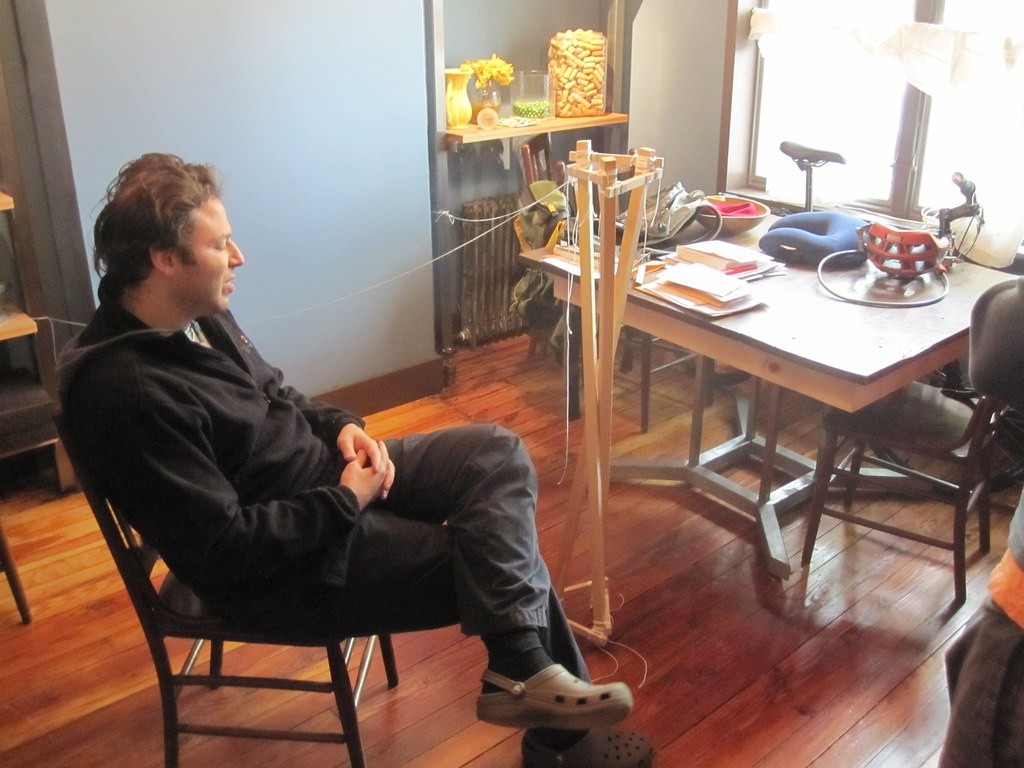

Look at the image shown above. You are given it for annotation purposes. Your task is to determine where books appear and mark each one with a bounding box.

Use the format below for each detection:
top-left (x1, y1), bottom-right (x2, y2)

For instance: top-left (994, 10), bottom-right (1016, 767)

top-left (542, 239), bottom-right (789, 316)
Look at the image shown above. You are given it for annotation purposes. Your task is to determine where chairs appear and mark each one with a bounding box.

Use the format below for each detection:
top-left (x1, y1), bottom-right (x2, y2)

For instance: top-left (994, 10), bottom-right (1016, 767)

top-left (53, 410), bottom-right (402, 768)
top-left (802, 278), bottom-right (1024, 601)
top-left (521, 132), bottom-right (715, 433)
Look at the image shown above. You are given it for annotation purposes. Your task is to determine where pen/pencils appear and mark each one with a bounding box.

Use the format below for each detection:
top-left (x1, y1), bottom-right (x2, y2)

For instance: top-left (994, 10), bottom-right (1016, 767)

top-left (722, 264), bottom-right (763, 274)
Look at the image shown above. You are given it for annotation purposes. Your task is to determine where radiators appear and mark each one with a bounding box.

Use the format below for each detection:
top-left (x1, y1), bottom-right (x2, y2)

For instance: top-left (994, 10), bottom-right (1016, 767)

top-left (456, 193), bottom-right (532, 349)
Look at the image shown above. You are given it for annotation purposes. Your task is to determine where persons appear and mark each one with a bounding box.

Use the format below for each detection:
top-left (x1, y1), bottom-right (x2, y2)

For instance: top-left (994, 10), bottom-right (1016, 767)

top-left (58, 153), bottom-right (654, 767)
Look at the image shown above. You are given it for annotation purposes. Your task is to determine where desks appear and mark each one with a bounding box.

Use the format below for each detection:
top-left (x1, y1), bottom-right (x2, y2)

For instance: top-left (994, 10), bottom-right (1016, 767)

top-left (518, 215), bottom-right (1023, 583)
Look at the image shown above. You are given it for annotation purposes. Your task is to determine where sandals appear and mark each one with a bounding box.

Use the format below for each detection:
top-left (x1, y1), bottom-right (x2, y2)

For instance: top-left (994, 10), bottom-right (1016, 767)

top-left (476, 663), bottom-right (633, 729)
top-left (522, 726), bottom-right (654, 768)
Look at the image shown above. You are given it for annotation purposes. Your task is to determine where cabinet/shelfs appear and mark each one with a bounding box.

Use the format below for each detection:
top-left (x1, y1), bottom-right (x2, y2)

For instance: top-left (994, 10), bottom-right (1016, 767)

top-left (0, 72), bottom-right (80, 492)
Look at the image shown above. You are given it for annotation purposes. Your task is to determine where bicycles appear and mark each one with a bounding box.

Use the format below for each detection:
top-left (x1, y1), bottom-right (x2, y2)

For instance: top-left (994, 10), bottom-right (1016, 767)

top-left (670, 140), bottom-right (1024, 489)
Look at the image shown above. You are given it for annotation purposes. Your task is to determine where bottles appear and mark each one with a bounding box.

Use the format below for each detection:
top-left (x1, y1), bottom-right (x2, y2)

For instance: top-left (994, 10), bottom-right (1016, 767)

top-left (445, 69), bottom-right (472, 128)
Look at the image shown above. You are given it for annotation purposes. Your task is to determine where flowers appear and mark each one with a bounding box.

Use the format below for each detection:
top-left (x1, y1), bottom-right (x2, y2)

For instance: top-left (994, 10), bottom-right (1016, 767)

top-left (460, 53), bottom-right (515, 90)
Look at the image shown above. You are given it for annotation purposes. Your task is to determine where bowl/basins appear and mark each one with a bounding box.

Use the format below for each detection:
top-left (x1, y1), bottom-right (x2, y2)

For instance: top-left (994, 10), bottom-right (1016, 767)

top-left (696, 195), bottom-right (771, 236)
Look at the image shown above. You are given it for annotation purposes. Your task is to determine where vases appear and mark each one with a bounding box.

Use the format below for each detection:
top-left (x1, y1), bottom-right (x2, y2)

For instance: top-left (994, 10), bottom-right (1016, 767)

top-left (445, 69), bottom-right (474, 130)
top-left (469, 77), bottom-right (503, 125)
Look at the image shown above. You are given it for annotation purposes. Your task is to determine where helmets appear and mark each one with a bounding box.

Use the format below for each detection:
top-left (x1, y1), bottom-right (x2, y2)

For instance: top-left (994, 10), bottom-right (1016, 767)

top-left (856, 221), bottom-right (951, 283)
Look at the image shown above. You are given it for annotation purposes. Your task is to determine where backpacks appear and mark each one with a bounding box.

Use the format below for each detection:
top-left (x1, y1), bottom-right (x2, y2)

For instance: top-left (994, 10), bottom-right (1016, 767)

top-left (510, 178), bottom-right (573, 327)
top-left (563, 182), bottom-right (722, 256)
